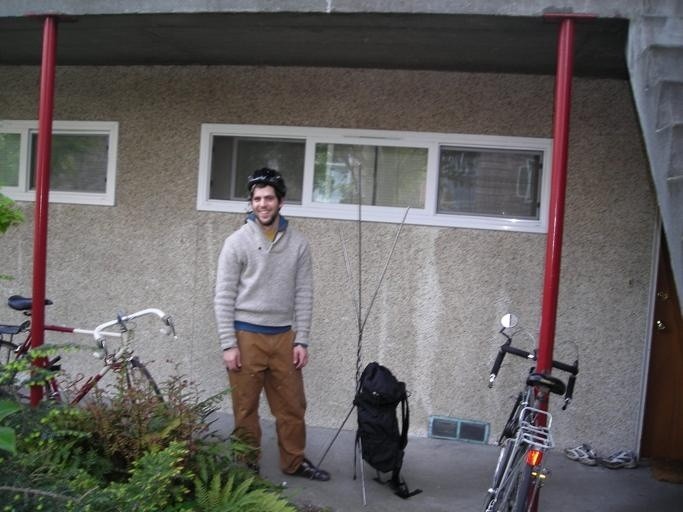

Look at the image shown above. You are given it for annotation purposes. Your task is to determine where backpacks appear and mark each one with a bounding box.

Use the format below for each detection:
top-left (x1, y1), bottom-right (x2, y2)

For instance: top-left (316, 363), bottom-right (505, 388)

top-left (353, 361), bottom-right (423, 498)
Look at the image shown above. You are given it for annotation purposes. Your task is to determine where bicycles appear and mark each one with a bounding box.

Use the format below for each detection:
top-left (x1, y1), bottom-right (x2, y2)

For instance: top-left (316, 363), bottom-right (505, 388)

top-left (0, 293), bottom-right (178, 419)
top-left (479, 314), bottom-right (581, 512)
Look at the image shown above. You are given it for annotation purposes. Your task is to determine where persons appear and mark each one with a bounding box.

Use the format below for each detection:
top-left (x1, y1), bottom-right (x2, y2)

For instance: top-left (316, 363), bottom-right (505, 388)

top-left (212, 166), bottom-right (331, 487)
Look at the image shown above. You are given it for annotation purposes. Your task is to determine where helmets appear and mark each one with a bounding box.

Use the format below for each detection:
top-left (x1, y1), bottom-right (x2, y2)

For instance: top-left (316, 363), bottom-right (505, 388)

top-left (246, 167), bottom-right (287, 198)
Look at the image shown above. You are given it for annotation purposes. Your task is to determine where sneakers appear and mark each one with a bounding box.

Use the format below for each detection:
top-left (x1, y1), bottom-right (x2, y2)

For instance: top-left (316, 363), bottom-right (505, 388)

top-left (564, 443), bottom-right (598, 467)
top-left (600, 448), bottom-right (638, 469)
top-left (289, 459), bottom-right (331, 482)
